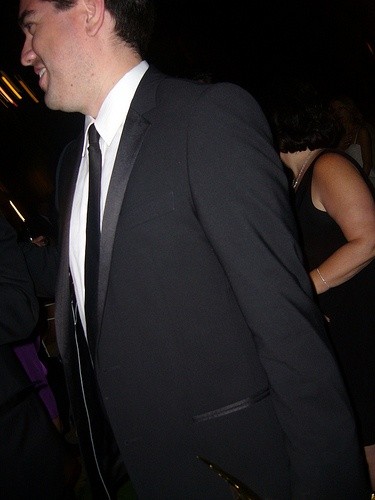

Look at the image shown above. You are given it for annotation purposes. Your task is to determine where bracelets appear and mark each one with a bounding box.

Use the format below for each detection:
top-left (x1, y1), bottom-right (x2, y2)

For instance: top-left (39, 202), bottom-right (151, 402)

top-left (317, 267), bottom-right (331, 289)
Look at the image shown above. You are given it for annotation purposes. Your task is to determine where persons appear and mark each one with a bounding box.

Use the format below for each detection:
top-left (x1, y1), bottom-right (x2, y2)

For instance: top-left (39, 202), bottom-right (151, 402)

top-left (0, 212), bottom-right (67, 500)
top-left (330, 90), bottom-right (375, 202)
top-left (23, 214), bottom-right (48, 247)
top-left (269, 79), bottom-right (375, 492)
top-left (21, 0), bottom-right (363, 500)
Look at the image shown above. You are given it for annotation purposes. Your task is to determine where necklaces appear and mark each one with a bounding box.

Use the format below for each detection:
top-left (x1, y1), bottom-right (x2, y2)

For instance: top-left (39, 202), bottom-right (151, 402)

top-left (290, 148), bottom-right (317, 189)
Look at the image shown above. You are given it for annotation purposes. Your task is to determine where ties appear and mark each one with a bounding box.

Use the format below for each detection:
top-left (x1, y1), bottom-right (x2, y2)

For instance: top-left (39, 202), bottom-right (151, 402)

top-left (84, 124), bottom-right (103, 361)
